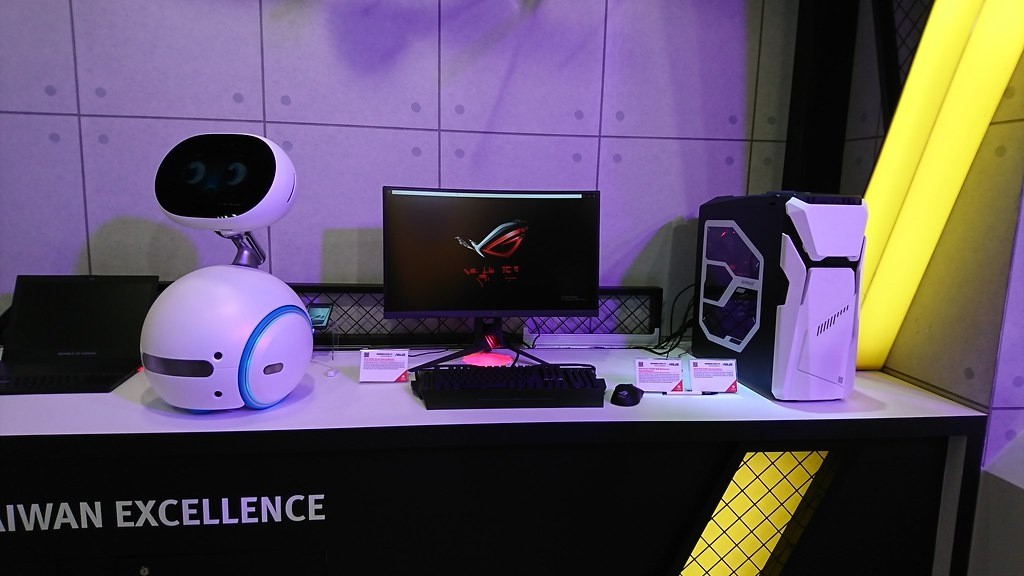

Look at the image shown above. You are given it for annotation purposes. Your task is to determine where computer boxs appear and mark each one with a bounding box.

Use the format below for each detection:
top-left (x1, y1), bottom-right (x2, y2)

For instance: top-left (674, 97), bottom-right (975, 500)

top-left (691, 191), bottom-right (868, 403)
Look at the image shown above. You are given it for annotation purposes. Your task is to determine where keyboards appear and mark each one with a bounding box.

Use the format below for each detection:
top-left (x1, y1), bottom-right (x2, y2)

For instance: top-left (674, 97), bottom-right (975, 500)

top-left (414, 368), bottom-right (604, 410)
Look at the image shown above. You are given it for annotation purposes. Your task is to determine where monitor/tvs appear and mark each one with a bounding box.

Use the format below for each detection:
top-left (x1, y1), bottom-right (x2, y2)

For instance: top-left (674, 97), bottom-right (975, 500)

top-left (382, 186), bottom-right (600, 371)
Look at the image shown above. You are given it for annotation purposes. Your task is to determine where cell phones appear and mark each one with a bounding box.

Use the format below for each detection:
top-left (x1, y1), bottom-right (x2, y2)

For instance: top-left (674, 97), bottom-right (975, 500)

top-left (308, 303), bottom-right (333, 330)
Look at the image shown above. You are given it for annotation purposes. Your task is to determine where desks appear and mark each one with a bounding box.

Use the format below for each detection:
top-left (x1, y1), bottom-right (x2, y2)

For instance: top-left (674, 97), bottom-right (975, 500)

top-left (0, 343), bottom-right (990, 576)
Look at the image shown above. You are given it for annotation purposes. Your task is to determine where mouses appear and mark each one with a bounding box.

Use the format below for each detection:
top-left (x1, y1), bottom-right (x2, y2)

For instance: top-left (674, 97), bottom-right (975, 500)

top-left (610, 384), bottom-right (645, 406)
